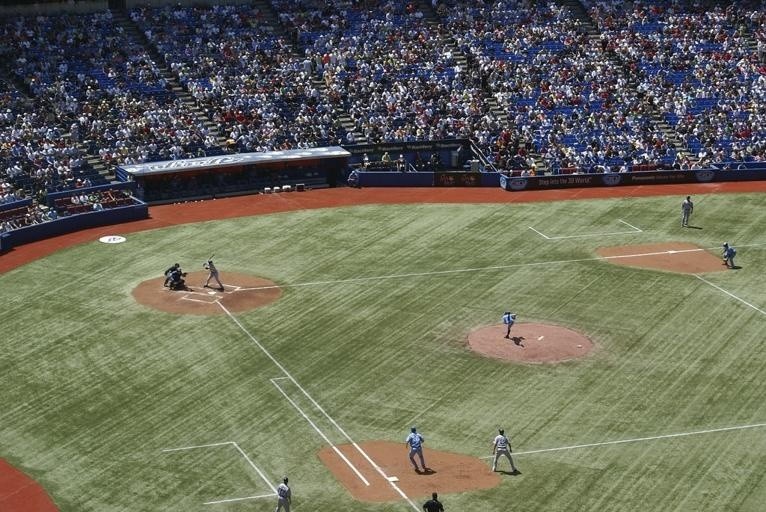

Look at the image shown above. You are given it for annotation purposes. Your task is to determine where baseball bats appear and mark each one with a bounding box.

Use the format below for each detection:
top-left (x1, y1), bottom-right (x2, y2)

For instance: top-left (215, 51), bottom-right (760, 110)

top-left (206, 254), bottom-right (215, 263)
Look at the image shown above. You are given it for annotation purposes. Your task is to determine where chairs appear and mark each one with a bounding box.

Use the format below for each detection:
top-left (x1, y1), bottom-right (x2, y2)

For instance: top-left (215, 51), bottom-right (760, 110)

top-left (1, 0), bottom-right (766, 235)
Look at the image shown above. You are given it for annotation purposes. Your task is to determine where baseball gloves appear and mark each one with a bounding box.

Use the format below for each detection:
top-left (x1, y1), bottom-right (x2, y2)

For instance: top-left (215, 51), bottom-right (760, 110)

top-left (182, 273), bottom-right (187, 277)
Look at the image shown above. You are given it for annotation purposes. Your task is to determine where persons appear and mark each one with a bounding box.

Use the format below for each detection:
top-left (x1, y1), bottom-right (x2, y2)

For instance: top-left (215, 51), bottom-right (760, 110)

top-left (164, 263), bottom-right (188, 286)
top-left (169, 267), bottom-right (187, 291)
top-left (276, 476), bottom-right (293, 512)
top-left (502, 311), bottom-right (518, 338)
top-left (422, 492), bottom-right (445, 512)
top-left (201, 260), bottom-right (224, 291)
top-left (721, 241), bottom-right (738, 270)
top-left (405, 426), bottom-right (430, 476)
top-left (492, 428), bottom-right (520, 474)
